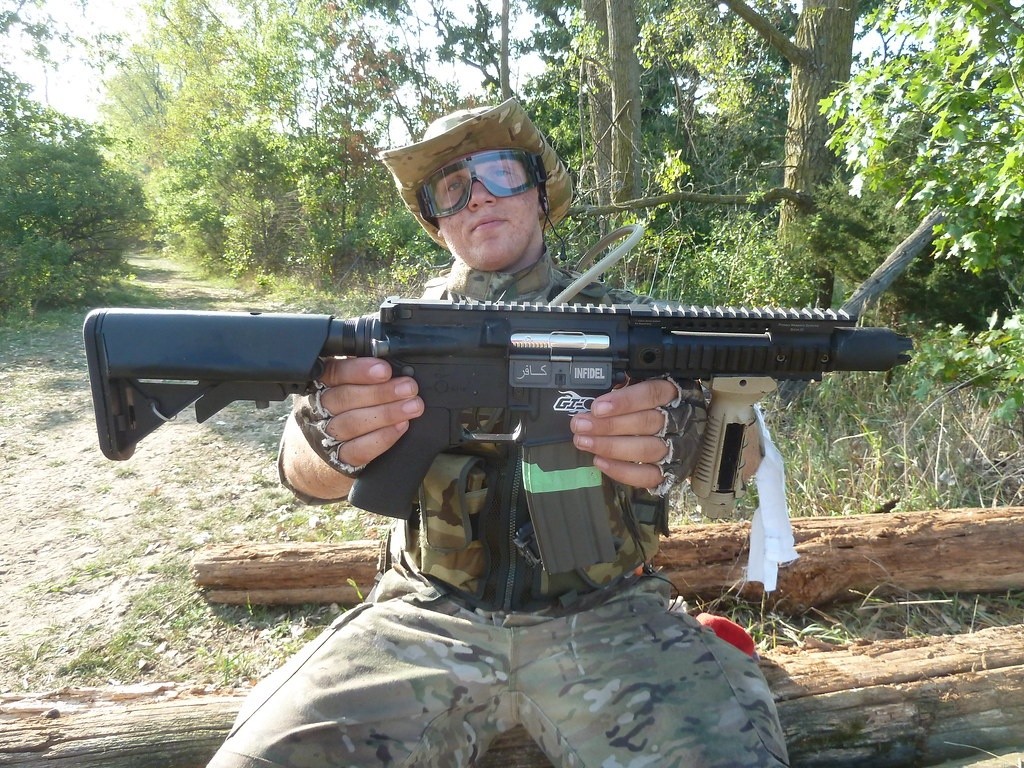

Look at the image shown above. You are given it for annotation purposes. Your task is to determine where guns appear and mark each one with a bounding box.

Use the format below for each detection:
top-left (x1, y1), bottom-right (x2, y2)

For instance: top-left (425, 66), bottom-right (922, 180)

top-left (83, 296), bottom-right (915, 577)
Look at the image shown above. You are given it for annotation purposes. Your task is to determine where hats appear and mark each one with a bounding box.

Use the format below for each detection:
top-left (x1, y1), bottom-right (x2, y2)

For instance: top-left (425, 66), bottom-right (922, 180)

top-left (377, 95), bottom-right (575, 254)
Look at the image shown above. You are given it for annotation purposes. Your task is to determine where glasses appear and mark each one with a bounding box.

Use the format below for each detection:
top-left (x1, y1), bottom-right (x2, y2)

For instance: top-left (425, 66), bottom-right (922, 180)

top-left (413, 145), bottom-right (549, 229)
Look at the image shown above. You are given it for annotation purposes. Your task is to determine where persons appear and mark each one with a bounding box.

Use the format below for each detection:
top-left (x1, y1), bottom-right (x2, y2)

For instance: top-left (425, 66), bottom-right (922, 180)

top-left (202, 97), bottom-right (790, 768)
